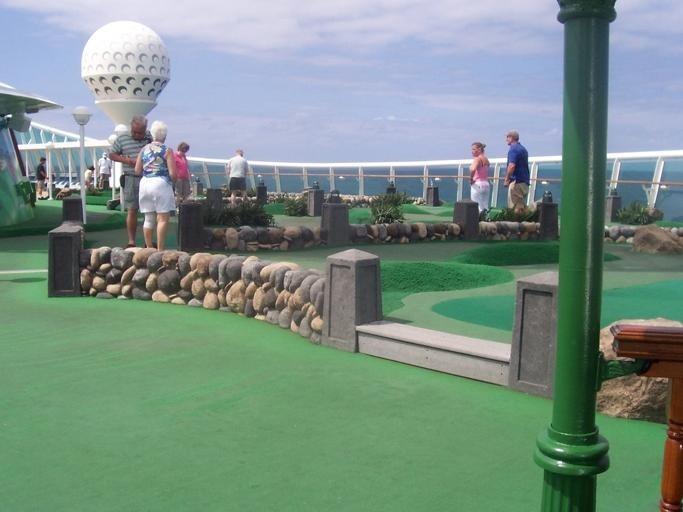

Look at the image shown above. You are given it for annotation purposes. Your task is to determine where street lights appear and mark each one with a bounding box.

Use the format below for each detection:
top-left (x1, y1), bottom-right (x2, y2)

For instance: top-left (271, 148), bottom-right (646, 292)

top-left (107, 136), bottom-right (116, 200)
top-left (46, 140), bottom-right (55, 201)
top-left (72, 107), bottom-right (92, 226)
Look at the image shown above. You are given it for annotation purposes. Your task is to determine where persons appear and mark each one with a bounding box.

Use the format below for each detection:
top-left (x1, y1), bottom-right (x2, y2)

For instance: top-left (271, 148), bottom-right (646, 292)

top-left (37, 157), bottom-right (47, 200)
top-left (470, 142), bottom-right (490, 221)
top-left (84, 151), bottom-right (112, 192)
top-left (226, 150), bottom-right (249, 203)
top-left (108, 115), bottom-right (192, 252)
top-left (504, 129), bottom-right (529, 214)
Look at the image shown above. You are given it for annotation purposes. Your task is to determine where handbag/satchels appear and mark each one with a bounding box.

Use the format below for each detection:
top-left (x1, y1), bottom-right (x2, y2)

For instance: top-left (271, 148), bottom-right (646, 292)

top-left (120, 174), bottom-right (126, 189)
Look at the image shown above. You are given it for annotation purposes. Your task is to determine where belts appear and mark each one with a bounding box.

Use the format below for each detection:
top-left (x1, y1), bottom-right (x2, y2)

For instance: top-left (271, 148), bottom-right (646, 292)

top-left (123, 173), bottom-right (141, 178)
